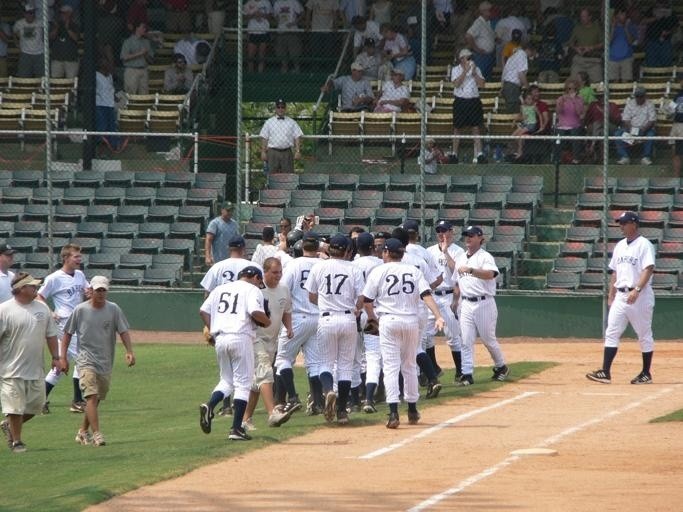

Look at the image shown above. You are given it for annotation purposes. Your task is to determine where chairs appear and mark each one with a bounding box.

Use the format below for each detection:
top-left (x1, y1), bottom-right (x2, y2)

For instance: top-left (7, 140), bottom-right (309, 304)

top-left (85, 269), bottom-right (112, 283)
top-left (9, 237), bottom-right (38, 253)
top-left (383, 191), bottom-right (414, 209)
top-left (247, 206), bottom-right (285, 223)
top-left (490, 113), bottom-right (525, 144)
top-left (668, 211), bottom-right (683, 225)
top-left (654, 258), bottom-right (679, 273)
top-left (544, 273), bottom-right (580, 290)
top-left (23, 108), bottom-right (59, 151)
top-left (60, 253), bottom-right (90, 266)
top-left (552, 257), bottom-right (587, 273)
top-left (507, 192), bottom-right (539, 235)
top-left (575, 193), bottom-right (611, 209)
top-left (31, 187), bottom-right (64, 204)
top-left (535, 80), bottom-right (565, 96)
top-left (112, 269), bottom-right (143, 285)
top-left (90, 253), bottom-right (120, 268)
top-left (407, 208), bottom-right (438, 226)
top-left (475, 225), bottom-right (493, 243)
top-left (564, 227), bottom-right (600, 244)
top-left (618, 177), bottom-right (648, 192)
top-left (648, 177), bottom-right (679, 192)
top-left (394, 111), bottom-right (427, 154)
top-left (165, 171), bottom-right (195, 187)
top-left (589, 258), bottom-right (612, 271)
top-left (469, 209), bottom-right (500, 226)
top-left (1, 109), bottom-right (26, 151)
top-left (187, 188), bottom-right (218, 218)
top-left (44, 76), bottom-right (78, 120)
top-left (10, 76), bottom-right (44, 94)
top-left (31, 92), bottom-right (69, 130)
top-left (264, 173), bottom-right (299, 189)
top-left (241, 222), bottom-right (277, 242)
top-left (107, 223), bottom-right (139, 237)
top-left (641, 65), bottom-right (676, 82)
top-left (257, 189), bottom-right (291, 206)
top-left (43, 222), bottom-right (76, 237)
top-left (658, 243), bottom-right (679, 256)
top-left (482, 176), bottom-right (512, 192)
top-left (244, 238), bottom-right (262, 255)
top-left (352, 190), bottom-right (384, 210)
top-left (0, 204), bottom-right (24, 222)
top-left (12, 222), bottom-right (44, 238)
top-left (134, 171), bottom-right (164, 185)
top-left (0, 170), bottom-right (13, 187)
top-left (391, 175), bottom-right (420, 193)
top-left (611, 194), bottom-right (642, 210)
top-left (328, 111), bottom-right (362, 154)
top-left (23, 253), bottom-right (59, 271)
top-left (22, 204), bottom-right (56, 222)
top-left (582, 177), bottom-right (617, 194)
top-left (291, 190), bottom-right (321, 208)
top-left (10, 254), bottom-right (25, 270)
top-left (408, 95), bottom-right (435, 113)
top-left (416, 192), bottom-right (444, 208)
top-left (72, 171), bottom-right (102, 187)
top-left (513, 176), bottom-right (544, 225)
top-left (132, 239), bottom-right (163, 253)
top-left (44, 171), bottom-right (73, 185)
top-left (581, 273), bottom-right (610, 287)
top-left (411, 77), bottom-right (443, 95)
top-left (642, 194), bottom-right (673, 210)
top-left (156, 95), bottom-right (190, 119)
top-left (155, 188), bottom-right (186, 204)
top-left (92, 187), bottom-right (126, 206)
top-left (493, 226), bottom-right (526, 259)
top-left (483, 112), bottom-right (491, 132)
top-left (300, 174), bottom-right (329, 190)
top-left (76, 222), bottom-right (107, 237)
top-left (662, 228), bottom-right (682, 243)
top-left (150, 110), bottom-right (180, 151)
top-left (139, 223), bottom-right (170, 239)
top-left (163, 238), bottom-right (194, 288)
top-left (362, 110), bottom-right (394, 155)
top-left (345, 208), bottom-right (375, 225)
top-left (117, 205), bottom-right (148, 221)
top-left (444, 193), bottom-right (475, 209)
top-left (426, 112), bottom-right (455, 139)
top-left (450, 175), bottom-right (481, 191)
top-left (0, 222), bottom-right (15, 238)
top-left (329, 173), bottom-right (359, 188)
top-left (607, 210), bottom-right (638, 227)
top-left (602, 80), bottom-right (636, 98)
top-left (1, 187), bottom-right (33, 204)
top-left (125, 93), bottom-right (158, 108)
top-left (178, 205), bottom-right (210, 238)
top-left (673, 194), bottom-right (683, 208)
top-left (103, 170), bottom-right (134, 186)
top-left (315, 208), bottom-right (345, 225)
top-left (118, 108), bottom-right (150, 140)
top-left (359, 175), bottom-right (390, 191)
top-left (638, 227), bottom-right (663, 243)
top-left (120, 254), bottom-right (152, 268)
top-left (486, 241), bottom-right (518, 285)
top-left (570, 210), bottom-right (603, 227)
top-left (195, 172), bottom-right (226, 204)
top-left (0, 75), bottom-right (12, 90)
top-left (376, 209), bottom-right (407, 225)
top-left (372, 225), bottom-right (398, 234)
top-left (494, 258), bottom-right (512, 287)
top-left (152, 255), bottom-right (185, 285)
top-left (601, 227), bottom-right (626, 238)
top-left (1, 92), bottom-right (36, 112)
top-left (13, 171), bottom-right (44, 188)
top-left (476, 192), bottom-right (506, 209)
top-left (37, 237), bottom-right (71, 253)
top-left (312, 225), bottom-right (338, 239)
top-left (499, 209), bottom-right (531, 252)
top-left (421, 175), bottom-right (450, 191)
top-left (144, 268), bottom-right (180, 288)
top-left (556, 243), bottom-right (593, 257)
top-left (439, 209), bottom-right (469, 225)
top-left (53, 206), bottom-right (86, 223)
top-left (61, 187), bottom-right (94, 206)
top-left (480, 95), bottom-right (498, 111)
top-left (72, 238), bottom-right (100, 253)
top-left (322, 190), bottom-right (352, 208)
top-left (171, 222), bottom-right (200, 267)
top-left (148, 206), bottom-right (179, 221)
top-left (639, 211), bottom-right (668, 229)
top-left (126, 186), bottom-right (155, 204)
top-left (340, 225), bottom-right (369, 236)
top-left (87, 206), bottom-right (116, 223)
top-left (635, 80), bottom-right (669, 99)
top-left (434, 96), bottom-right (455, 111)
top-left (594, 243), bottom-right (615, 253)
top-left (418, 63), bottom-right (449, 80)
top-left (285, 208), bottom-right (314, 224)
top-left (101, 239), bottom-right (132, 254)
top-left (651, 274), bottom-right (678, 292)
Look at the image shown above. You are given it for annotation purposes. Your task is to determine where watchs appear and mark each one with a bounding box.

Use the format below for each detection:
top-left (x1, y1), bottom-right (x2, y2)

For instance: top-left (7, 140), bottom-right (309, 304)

top-left (634, 286), bottom-right (641, 292)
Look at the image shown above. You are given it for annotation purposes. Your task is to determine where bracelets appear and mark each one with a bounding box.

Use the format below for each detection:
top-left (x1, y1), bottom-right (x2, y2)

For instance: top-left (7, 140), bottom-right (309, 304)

top-left (469, 267), bottom-right (474, 275)
top-left (52, 355), bottom-right (60, 361)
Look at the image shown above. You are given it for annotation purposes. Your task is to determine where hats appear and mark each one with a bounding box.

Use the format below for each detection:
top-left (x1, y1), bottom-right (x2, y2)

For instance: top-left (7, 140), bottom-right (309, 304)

top-left (13, 275), bottom-right (41, 288)
top-left (91, 276), bottom-right (109, 291)
top-left (238, 266), bottom-right (262, 278)
top-left (616, 212), bottom-right (639, 223)
top-left (303, 232), bottom-right (319, 242)
top-left (222, 201), bottom-right (236, 209)
top-left (403, 221), bottom-right (419, 234)
top-left (351, 62), bottom-right (365, 71)
top-left (229, 236), bottom-right (245, 247)
top-left (356, 233), bottom-right (374, 247)
top-left (0, 244), bottom-right (15, 255)
top-left (382, 238), bottom-right (402, 251)
top-left (461, 226), bottom-right (483, 236)
top-left (435, 220), bottom-right (453, 229)
top-left (23, 4), bottom-right (38, 12)
top-left (330, 235), bottom-right (348, 249)
top-left (277, 98), bottom-right (285, 105)
top-left (635, 86), bottom-right (646, 95)
top-left (365, 38), bottom-right (375, 45)
top-left (459, 49), bottom-right (472, 58)
top-left (592, 82), bottom-right (605, 95)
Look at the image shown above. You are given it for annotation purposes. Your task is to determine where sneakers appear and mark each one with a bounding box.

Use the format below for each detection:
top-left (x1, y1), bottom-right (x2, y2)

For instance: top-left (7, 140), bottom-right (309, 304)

top-left (408, 408), bottom-right (420, 425)
top-left (1, 419), bottom-right (8, 437)
top-left (386, 412), bottom-right (400, 428)
top-left (323, 391), bottom-right (336, 423)
top-left (76, 430), bottom-right (92, 444)
top-left (352, 403), bottom-right (361, 413)
top-left (200, 402), bottom-right (214, 434)
top-left (241, 418), bottom-right (257, 431)
top-left (283, 393), bottom-right (302, 415)
top-left (458, 375), bottom-right (474, 386)
top-left (642, 157), bottom-right (653, 165)
top-left (421, 365), bottom-right (444, 386)
top-left (216, 407), bottom-right (232, 417)
top-left (14, 444), bottom-right (27, 452)
top-left (426, 381), bottom-right (442, 399)
top-left (363, 399), bottom-right (375, 413)
top-left (338, 411), bottom-right (350, 423)
top-left (41, 402), bottom-right (50, 415)
top-left (229, 427), bottom-right (251, 440)
top-left (631, 372), bottom-right (652, 384)
top-left (617, 157), bottom-right (630, 165)
top-left (493, 366), bottom-right (510, 381)
top-left (587, 369), bottom-right (610, 384)
top-left (70, 399), bottom-right (85, 413)
top-left (446, 155), bottom-right (458, 164)
top-left (268, 408), bottom-right (290, 427)
top-left (477, 155), bottom-right (485, 162)
top-left (92, 432), bottom-right (105, 446)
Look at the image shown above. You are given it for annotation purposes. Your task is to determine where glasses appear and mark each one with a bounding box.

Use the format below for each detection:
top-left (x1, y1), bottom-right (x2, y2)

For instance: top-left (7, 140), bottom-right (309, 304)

top-left (281, 224), bottom-right (289, 228)
top-left (436, 228), bottom-right (447, 233)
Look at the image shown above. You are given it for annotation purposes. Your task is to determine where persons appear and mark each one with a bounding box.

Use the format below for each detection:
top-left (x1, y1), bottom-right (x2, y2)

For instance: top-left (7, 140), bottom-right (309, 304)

top-left (163, 0), bottom-right (193, 35)
top-left (201, 1), bottom-right (226, 35)
top-left (494, 6), bottom-right (529, 45)
top-left (502, 30), bottom-right (524, 64)
top-left (614, 87), bottom-right (658, 166)
top-left (609, 7), bottom-right (640, 83)
top-left (466, 1), bottom-right (503, 83)
top-left (424, 137), bottom-right (447, 165)
top-left (240, 257), bottom-right (291, 432)
top-left (584, 83), bottom-right (623, 165)
top-left (199, 266), bottom-right (271, 441)
top-left (497, 84), bottom-right (550, 166)
top-left (0, 272), bottom-right (59, 453)
top-left (274, 233), bottom-right (325, 415)
top-left (200, 234), bottom-right (265, 301)
top-left (447, 49), bottom-right (489, 164)
top-left (303, 233), bottom-right (365, 425)
top-left (119, 19), bottom-right (155, 95)
top-left (59, 278), bottom-right (135, 447)
top-left (420, 221), bottom-right (466, 386)
top-left (12, 3), bottom-right (45, 78)
top-left (567, 7), bottom-right (604, 84)
top-left (537, 27), bottom-right (566, 84)
top-left (0, 21), bottom-right (20, 78)
top-left (250, 226), bottom-right (293, 271)
top-left (241, 0), bottom-right (453, 79)
top-left (200, 236), bottom-right (264, 418)
top-left (374, 66), bottom-right (411, 113)
top-left (398, 219), bottom-right (443, 291)
top-left (349, 232), bottom-right (384, 414)
top-left (573, 72), bottom-right (598, 104)
top-left (392, 227), bottom-right (442, 399)
top-left (375, 231), bottom-right (391, 260)
top-left (586, 211), bottom-right (655, 384)
top-left (449, 226), bottom-right (511, 387)
top-left (500, 41), bottom-right (537, 114)
top-left (551, 78), bottom-right (586, 165)
top-left (0, 243), bottom-right (16, 305)
top-left (49, 5), bottom-right (80, 79)
top-left (278, 217), bottom-right (292, 249)
top-left (319, 62), bottom-right (375, 113)
top-left (36, 243), bottom-right (92, 415)
top-left (164, 52), bottom-right (193, 95)
top-left (362, 238), bottom-right (446, 428)
top-left (94, 59), bottom-right (128, 156)
top-left (259, 98), bottom-right (304, 174)
top-left (644, 5), bottom-right (678, 68)
